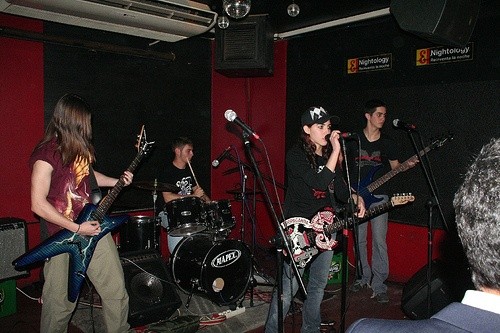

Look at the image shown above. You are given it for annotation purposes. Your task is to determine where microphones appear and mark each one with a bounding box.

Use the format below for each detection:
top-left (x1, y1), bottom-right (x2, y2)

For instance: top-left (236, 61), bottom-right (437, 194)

top-left (212, 146), bottom-right (232, 167)
top-left (393, 119), bottom-right (417, 132)
top-left (325, 133), bottom-right (352, 141)
top-left (225, 110), bottom-right (262, 141)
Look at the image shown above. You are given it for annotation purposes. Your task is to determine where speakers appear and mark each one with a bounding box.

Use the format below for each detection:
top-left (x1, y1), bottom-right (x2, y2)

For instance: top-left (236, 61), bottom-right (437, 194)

top-left (401, 258), bottom-right (470, 321)
top-left (0, 217), bottom-right (30, 281)
top-left (117, 248), bottom-right (182, 328)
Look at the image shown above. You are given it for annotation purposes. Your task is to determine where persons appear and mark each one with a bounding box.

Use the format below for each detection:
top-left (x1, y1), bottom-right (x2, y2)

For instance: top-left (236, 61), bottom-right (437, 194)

top-left (27, 91), bottom-right (134, 333)
top-left (345, 139), bottom-right (500, 333)
top-left (153, 137), bottom-right (210, 224)
top-left (264, 100), bottom-right (420, 333)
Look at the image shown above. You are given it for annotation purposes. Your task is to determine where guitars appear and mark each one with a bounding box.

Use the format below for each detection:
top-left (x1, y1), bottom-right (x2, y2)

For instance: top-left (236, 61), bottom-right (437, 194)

top-left (12, 124), bottom-right (155, 304)
top-left (279, 192), bottom-right (416, 268)
top-left (349, 131), bottom-right (454, 213)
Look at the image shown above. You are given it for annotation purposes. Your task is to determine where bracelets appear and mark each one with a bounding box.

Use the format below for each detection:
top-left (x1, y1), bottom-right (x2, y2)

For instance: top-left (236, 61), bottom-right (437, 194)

top-left (76, 224), bottom-right (80, 234)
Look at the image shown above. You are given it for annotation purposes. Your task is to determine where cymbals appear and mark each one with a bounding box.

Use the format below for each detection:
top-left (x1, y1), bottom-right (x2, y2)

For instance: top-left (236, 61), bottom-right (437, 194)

top-left (225, 188), bottom-right (262, 195)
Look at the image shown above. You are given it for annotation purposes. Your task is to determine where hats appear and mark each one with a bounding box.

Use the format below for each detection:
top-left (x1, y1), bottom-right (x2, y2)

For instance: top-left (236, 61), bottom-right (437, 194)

top-left (301, 106), bottom-right (341, 125)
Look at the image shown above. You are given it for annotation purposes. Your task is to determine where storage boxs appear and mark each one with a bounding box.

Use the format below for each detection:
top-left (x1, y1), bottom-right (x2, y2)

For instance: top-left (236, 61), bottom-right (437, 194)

top-left (0, 278), bottom-right (18, 318)
top-left (301, 252), bottom-right (347, 285)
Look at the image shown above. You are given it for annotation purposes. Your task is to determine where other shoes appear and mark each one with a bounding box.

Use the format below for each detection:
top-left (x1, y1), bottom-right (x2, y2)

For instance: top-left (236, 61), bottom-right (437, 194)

top-left (349, 281), bottom-right (367, 292)
top-left (374, 291), bottom-right (389, 303)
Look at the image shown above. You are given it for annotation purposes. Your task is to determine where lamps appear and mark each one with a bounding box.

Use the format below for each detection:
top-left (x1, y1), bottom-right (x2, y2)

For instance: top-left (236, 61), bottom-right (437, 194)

top-left (287, 0), bottom-right (300, 17)
top-left (223, 0), bottom-right (251, 19)
top-left (274, 7), bottom-right (392, 42)
top-left (218, 16), bottom-right (229, 28)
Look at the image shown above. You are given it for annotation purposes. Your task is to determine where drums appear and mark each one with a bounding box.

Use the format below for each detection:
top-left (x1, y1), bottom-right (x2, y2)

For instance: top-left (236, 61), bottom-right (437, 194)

top-left (202, 197), bottom-right (240, 235)
top-left (169, 230), bottom-right (253, 306)
top-left (164, 194), bottom-right (209, 238)
top-left (115, 216), bottom-right (163, 264)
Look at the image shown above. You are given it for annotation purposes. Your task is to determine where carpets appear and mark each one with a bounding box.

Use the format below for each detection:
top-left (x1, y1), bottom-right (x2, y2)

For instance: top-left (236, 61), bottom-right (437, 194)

top-left (70, 280), bottom-right (303, 333)
